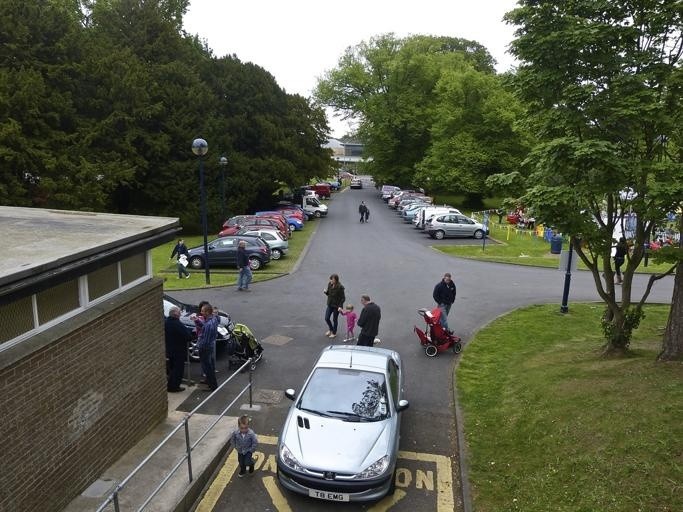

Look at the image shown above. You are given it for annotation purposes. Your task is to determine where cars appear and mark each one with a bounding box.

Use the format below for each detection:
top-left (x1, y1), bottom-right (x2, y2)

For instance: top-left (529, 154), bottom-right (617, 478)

top-left (350, 179), bottom-right (362, 189)
top-left (379, 185), bottom-right (489, 240)
top-left (275, 345), bottom-right (410, 502)
top-left (185, 182), bottom-right (340, 271)
top-left (163, 292), bottom-right (232, 361)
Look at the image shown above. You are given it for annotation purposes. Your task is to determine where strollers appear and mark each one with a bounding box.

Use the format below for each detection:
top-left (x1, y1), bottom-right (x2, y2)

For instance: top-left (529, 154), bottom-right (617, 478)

top-left (222, 323), bottom-right (262, 371)
top-left (414, 308), bottom-right (462, 357)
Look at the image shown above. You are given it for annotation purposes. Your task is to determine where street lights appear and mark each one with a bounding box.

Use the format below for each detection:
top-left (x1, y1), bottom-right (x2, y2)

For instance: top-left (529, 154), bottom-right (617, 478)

top-left (218, 157), bottom-right (228, 223)
top-left (191, 138), bottom-right (209, 283)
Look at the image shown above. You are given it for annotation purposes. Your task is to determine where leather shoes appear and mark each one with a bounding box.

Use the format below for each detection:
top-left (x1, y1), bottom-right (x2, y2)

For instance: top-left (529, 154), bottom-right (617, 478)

top-left (168, 388), bottom-right (185, 392)
top-left (200, 379), bottom-right (215, 391)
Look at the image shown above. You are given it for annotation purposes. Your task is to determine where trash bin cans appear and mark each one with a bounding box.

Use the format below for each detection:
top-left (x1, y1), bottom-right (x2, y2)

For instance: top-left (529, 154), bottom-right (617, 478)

top-left (551, 236), bottom-right (563, 254)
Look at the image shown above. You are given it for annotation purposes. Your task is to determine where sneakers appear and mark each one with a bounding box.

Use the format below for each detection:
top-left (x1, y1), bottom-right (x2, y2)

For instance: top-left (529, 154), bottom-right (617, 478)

top-left (348, 336), bottom-right (356, 341)
top-left (249, 465), bottom-right (253, 473)
top-left (236, 287), bottom-right (251, 292)
top-left (239, 467), bottom-right (247, 475)
top-left (343, 338), bottom-right (348, 342)
top-left (329, 333), bottom-right (336, 338)
top-left (186, 273), bottom-right (191, 278)
top-left (325, 330), bottom-right (332, 336)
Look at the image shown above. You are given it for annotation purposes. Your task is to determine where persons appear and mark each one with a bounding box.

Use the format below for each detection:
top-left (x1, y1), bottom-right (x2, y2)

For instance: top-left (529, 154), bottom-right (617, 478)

top-left (359, 201), bottom-right (370, 224)
top-left (236, 241), bottom-right (253, 292)
top-left (323, 274), bottom-right (345, 338)
top-left (189, 301), bottom-right (220, 392)
top-left (433, 273), bottom-right (456, 330)
top-left (613, 238), bottom-right (627, 284)
top-left (340, 305), bottom-right (359, 342)
top-left (356, 295), bottom-right (381, 347)
top-left (231, 416), bottom-right (257, 478)
top-left (169, 239), bottom-right (192, 279)
top-left (165, 307), bottom-right (195, 393)
